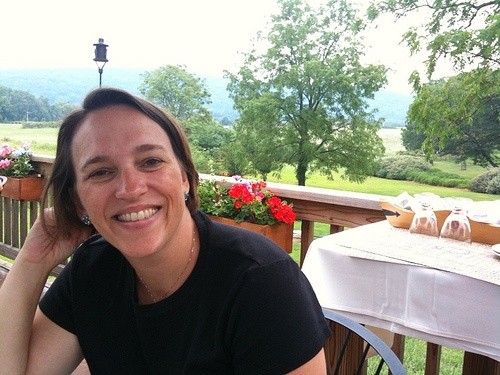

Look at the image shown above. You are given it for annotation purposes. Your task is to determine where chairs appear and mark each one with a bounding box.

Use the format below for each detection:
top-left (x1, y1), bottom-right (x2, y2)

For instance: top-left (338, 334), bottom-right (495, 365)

top-left (315, 308), bottom-right (406, 375)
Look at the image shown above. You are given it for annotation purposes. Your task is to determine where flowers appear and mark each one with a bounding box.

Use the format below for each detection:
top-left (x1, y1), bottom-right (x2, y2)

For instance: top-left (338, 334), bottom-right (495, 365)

top-left (0, 143), bottom-right (34, 177)
top-left (196, 175), bottom-right (297, 225)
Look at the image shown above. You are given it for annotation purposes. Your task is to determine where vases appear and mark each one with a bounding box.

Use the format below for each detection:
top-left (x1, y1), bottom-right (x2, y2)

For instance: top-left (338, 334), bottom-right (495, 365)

top-left (2, 175), bottom-right (45, 200)
top-left (207, 216), bottom-right (293, 256)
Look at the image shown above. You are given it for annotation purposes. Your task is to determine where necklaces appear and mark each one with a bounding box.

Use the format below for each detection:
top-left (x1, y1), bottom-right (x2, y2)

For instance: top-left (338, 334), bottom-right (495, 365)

top-left (133, 226), bottom-right (197, 304)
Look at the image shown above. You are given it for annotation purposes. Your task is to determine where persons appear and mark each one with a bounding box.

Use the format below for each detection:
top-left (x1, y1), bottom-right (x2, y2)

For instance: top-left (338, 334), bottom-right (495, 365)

top-left (0, 87), bottom-right (333, 375)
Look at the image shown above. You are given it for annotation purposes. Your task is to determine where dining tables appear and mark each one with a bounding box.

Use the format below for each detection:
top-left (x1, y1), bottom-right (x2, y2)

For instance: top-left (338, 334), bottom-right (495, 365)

top-left (301, 219), bottom-right (499, 374)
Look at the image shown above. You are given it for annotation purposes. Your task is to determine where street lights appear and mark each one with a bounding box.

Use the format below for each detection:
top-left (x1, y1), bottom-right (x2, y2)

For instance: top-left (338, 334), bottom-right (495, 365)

top-left (93, 37), bottom-right (109, 88)
